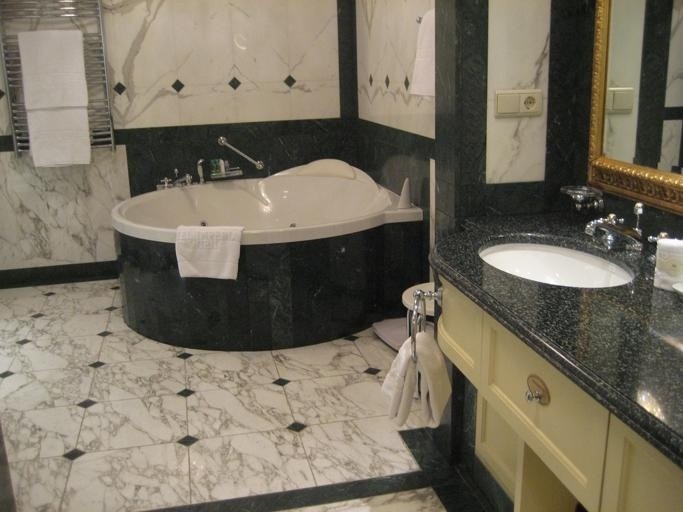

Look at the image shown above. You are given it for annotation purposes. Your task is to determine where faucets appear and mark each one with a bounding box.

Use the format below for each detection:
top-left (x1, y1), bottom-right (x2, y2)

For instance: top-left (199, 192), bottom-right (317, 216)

top-left (196, 158), bottom-right (206, 183)
top-left (583, 220), bottom-right (648, 249)
top-left (174, 173), bottom-right (194, 186)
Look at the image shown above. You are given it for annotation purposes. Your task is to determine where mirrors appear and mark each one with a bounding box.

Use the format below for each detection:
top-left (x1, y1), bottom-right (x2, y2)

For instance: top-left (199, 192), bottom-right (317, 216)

top-left (587, 1), bottom-right (681, 216)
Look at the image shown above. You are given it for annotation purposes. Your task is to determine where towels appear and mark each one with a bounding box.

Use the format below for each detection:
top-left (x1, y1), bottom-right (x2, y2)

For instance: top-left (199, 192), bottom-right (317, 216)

top-left (172, 222), bottom-right (241, 280)
top-left (382, 330), bottom-right (452, 430)
top-left (409, 7), bottom-right (434, 100)
top-left (14, 28), bottom-right (90, 168)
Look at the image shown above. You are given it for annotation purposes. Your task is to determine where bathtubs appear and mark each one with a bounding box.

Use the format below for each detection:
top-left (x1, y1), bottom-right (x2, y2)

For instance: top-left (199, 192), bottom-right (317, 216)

top-left (110, 159), bottom-right (423, 348)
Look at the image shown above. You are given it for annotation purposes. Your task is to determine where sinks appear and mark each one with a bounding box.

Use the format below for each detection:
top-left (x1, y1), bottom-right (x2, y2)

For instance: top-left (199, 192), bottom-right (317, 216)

top-left (477, 233), bottom-right (635, 290)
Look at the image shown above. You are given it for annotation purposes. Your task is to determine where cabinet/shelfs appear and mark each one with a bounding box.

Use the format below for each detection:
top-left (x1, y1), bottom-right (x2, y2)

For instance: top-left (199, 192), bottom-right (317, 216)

top-left (481, 315), bottom-right (682, 510)
